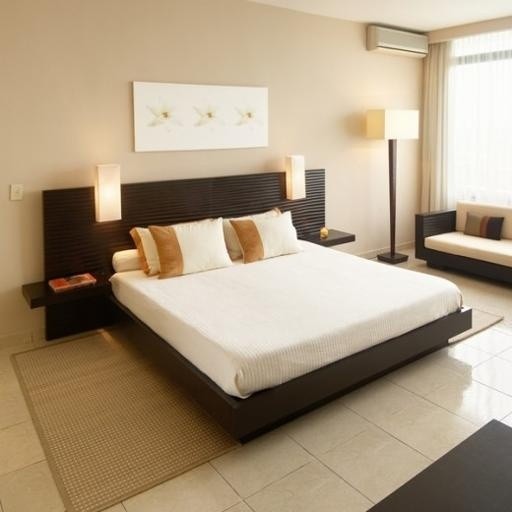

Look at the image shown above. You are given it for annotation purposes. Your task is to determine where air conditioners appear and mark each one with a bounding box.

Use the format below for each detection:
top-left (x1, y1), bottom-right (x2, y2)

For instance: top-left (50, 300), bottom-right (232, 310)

top-left (367, 25), bottom-right (428, 60)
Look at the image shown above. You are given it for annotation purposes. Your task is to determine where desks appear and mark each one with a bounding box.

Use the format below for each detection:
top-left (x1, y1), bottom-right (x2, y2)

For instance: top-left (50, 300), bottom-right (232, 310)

top-left (300, 228), bottom-right (355, 247)
top-left (21, 267), bottom-right (111, 308)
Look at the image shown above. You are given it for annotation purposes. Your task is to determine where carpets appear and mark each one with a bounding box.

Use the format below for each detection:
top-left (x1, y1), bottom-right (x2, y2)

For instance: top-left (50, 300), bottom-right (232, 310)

top-left (11, 306), bottom-right (505, 512)
top-left (365, 417), bottom-right (512, 511)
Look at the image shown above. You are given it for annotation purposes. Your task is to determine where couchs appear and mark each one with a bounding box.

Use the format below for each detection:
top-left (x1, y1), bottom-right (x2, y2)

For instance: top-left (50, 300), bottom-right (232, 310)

top-left (414, 201), bottom-right (511, 288)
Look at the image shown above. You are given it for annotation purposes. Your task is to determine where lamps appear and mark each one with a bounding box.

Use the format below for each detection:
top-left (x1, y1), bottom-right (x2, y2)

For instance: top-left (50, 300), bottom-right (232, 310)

top-left (286, 155), bottom-right (306, 202)
top-left (366, 107), bottom-right (418, 263)
top-left (94, 164), bottom-right (122, 225)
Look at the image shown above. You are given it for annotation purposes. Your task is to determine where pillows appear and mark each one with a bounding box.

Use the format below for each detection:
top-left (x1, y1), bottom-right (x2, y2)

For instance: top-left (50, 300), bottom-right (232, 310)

top-left (222, 207), bottom-right (281, 262)
top-left (127, 217), bottom-right (216, 278)
top-left (463, 211), bottom-right (504, 240)
top-left (111, 248), bottom-right (143, 272)
top-left (230, 211), bottom-right (299, 264)
top-left (148, 217), bottom-right (233, 281)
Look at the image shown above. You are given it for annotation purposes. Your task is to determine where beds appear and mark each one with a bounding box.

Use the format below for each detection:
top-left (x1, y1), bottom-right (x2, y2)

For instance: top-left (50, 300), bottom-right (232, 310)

top-left (42, 170), bottom-right (472, 444)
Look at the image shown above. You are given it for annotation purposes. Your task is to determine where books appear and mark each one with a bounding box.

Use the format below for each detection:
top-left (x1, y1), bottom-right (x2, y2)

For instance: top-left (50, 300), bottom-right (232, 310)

top-left (48, 273), bottom-right (96, 293)
